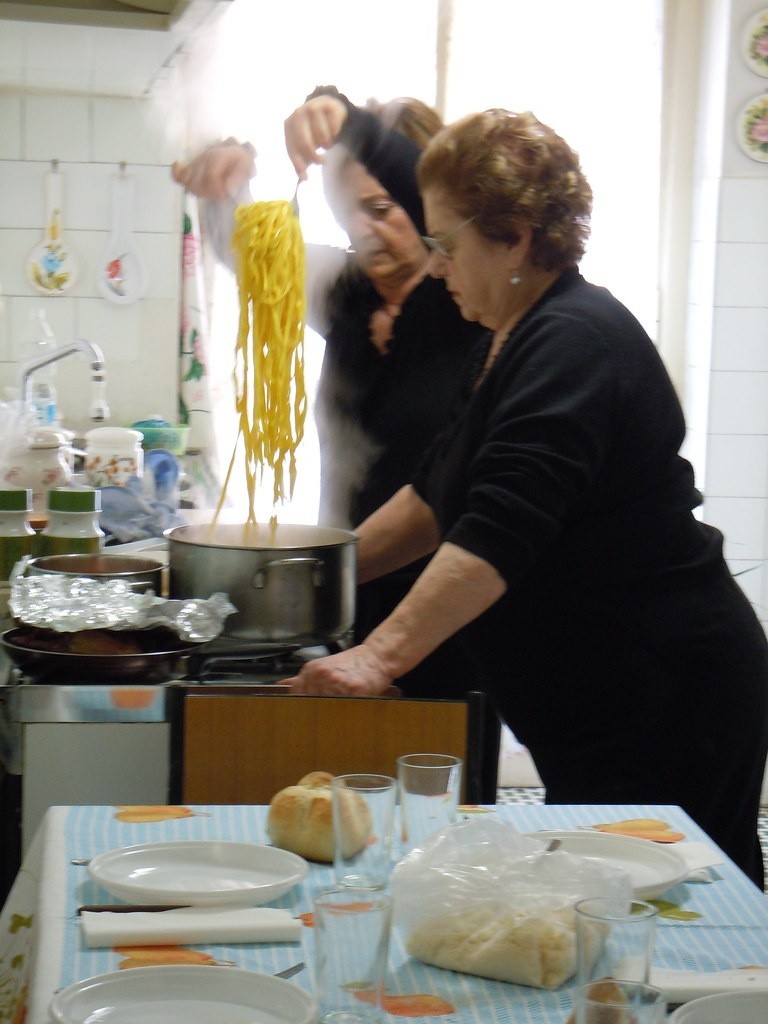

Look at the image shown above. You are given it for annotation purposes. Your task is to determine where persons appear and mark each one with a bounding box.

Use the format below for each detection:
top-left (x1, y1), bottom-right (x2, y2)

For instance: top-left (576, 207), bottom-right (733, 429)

top-left (277, 109), bottom-right (768, 892)
top-left (171, 84), bottom-right (503, 803)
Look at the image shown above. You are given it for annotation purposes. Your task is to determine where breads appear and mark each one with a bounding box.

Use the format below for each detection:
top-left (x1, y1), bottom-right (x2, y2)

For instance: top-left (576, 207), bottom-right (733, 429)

top-left (266, 770), bottom-right (372, 865)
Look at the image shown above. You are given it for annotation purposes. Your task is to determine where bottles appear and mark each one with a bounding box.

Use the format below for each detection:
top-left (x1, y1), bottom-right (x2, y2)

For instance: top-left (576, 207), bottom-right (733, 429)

top-left (0, 384), bottom-right (143, 588)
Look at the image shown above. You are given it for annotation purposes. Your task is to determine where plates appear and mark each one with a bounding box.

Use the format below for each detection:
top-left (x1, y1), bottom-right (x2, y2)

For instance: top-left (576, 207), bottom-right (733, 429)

top-left (524, 831), bottom-right (688, 901)
top-left (89, 842), bottom-right (307, 907)
top-left (48, 965), bottom-right (313, 1024)
top-left (668, 991), bottom-right (768, 1024)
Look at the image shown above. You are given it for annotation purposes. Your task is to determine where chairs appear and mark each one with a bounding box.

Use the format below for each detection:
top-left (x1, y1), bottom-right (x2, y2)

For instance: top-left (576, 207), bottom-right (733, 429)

top-left (171, 688), bottom-right (504, 805)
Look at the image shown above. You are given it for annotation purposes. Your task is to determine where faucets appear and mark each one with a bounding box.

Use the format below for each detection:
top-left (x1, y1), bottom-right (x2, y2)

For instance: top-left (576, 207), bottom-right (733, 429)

top-left (22, 341), bottom-right (110, 440)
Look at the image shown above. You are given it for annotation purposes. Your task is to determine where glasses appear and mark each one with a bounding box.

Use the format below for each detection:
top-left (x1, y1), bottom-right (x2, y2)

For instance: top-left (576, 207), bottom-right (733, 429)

top-left (419, 212), bottom-right (542, 262)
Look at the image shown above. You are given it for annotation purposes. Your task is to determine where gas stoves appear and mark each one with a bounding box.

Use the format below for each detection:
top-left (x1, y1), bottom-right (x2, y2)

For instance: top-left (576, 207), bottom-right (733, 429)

top-left (18, 601), bottom-right (364, 691)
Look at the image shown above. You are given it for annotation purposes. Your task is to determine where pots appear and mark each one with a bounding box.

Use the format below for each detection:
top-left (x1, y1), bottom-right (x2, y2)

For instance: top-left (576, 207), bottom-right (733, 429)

top-left (25, 555), bottom-right (168, 619)
top-left (0, 626), bottom-right (302, 683)
top-left (162, 523), bottom-right (360, 647)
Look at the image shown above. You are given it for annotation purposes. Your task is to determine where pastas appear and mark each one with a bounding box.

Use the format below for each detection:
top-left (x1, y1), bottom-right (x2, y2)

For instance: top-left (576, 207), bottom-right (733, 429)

top-left (198, 199), bottom-right (310, 548)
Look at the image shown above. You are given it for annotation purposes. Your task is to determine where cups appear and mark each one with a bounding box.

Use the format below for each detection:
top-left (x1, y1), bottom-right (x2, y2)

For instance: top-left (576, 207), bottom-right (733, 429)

top-left (574, 981), bottom-right (665, 1024)
top-left (331, 774), bottom-right (397, 891)
top-left (574, 898), bottom-right (660, 985)
top-left (398, 755), bottom-right (463, 856)
top-left (315, 893), bottom-right (392, 1024)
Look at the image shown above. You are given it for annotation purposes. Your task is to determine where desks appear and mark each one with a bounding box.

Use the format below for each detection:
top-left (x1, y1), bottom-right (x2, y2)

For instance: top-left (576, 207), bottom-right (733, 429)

top-left (0, 803), bottom-right (766, 1023)
